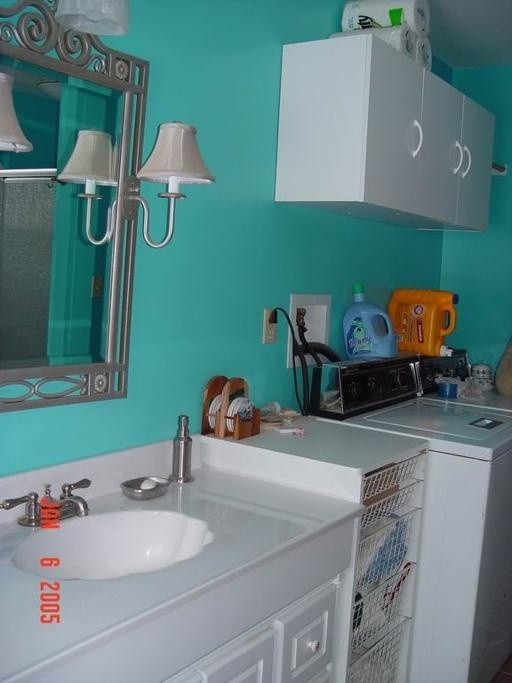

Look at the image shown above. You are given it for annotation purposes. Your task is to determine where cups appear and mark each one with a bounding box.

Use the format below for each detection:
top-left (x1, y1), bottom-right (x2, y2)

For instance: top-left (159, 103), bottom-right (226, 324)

top-left (464, 377), bottom-right (482, 396)
top-left (478, 378), bottom-right (494, 392)
top-left (471, 364), bottom-right (491, 378)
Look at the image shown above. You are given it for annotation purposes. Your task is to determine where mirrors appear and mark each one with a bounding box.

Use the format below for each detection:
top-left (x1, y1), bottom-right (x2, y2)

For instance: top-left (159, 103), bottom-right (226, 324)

top-left (0, 58), bottom-right (123, 374)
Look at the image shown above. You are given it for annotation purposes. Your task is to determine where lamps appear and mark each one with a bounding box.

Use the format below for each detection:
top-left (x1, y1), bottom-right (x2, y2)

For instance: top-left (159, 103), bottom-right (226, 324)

top-left (56, 127), bottom-right (118, 245)
top-left (124, 122), bottom-right (214, 248)
top-left (51, 0), bottom-right (130, 36)
top-left (0, 70), bottom-right (34, 153)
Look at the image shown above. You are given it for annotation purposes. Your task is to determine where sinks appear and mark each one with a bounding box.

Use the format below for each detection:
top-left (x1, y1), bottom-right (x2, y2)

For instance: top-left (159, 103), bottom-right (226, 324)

top-left (10, 511), bottom-right (213, 580)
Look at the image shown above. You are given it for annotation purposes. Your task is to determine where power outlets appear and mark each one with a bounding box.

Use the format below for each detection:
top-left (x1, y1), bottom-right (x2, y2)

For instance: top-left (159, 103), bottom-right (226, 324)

top-left (263, 309), bottom-right (276, 342)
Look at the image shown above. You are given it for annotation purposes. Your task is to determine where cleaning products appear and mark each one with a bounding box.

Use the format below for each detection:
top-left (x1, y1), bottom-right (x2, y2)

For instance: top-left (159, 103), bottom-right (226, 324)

top-left (341, 283), bottom-right (396, 358)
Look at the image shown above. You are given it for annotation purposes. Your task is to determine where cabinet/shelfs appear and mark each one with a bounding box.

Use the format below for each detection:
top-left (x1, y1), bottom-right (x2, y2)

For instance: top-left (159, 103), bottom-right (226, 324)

top-left (276, 34), bottom-right (420, 223)
top-left (421, 66), bottom-right (499, 232)
top-left (160, 572), bottom-right (355, 683)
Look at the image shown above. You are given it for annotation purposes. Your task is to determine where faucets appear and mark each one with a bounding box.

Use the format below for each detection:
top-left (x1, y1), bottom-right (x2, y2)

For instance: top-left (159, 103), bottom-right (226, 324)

top-left (1, 478), bottom-right (91, 529)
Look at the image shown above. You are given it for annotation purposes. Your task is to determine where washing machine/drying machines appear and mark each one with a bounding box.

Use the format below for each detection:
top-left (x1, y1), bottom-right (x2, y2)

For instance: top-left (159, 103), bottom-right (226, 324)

top-left (409, 351), bottom-right (511, 410)
top-left (308, 359), bottom-right (510, 683)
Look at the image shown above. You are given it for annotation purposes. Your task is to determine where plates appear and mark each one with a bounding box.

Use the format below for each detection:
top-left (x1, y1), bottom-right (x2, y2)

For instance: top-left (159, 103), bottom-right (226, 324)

top-left (207, 395), bottom-right (221, 429)
top-left (226, 396), bottom-right (255, 432)
